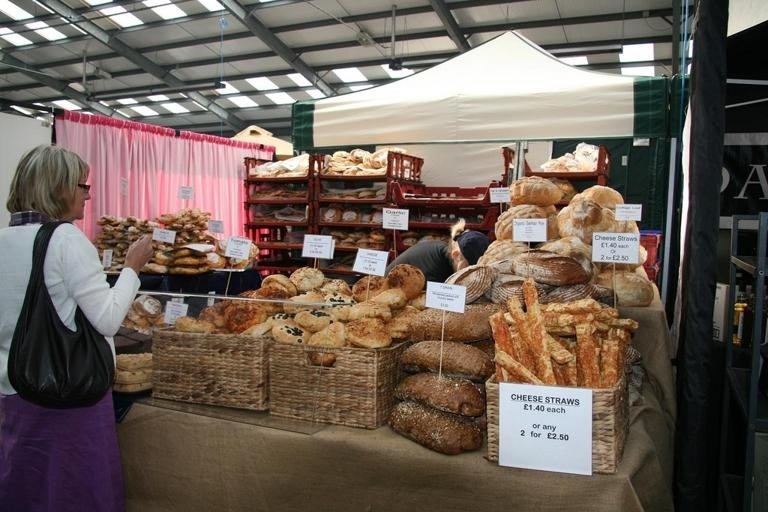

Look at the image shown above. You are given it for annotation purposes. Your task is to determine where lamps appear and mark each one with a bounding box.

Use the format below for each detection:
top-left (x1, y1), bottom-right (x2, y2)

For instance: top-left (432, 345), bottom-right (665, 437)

top-left (83, 36), bottom-right (226, 103)
top-left (389, 0), bottom-right (623, 70)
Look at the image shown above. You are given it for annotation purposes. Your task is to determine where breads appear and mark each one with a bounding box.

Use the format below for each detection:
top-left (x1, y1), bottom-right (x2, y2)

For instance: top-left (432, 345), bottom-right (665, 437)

top-left (171, 262), bottom-right (428, 366)
top-left (251, 147), bottom-right (407, 248)
top-left (110, 353), bottom-right (152, 393)
top-left (488, 277), bottom-right (640, 388)
top-left (478, 175), bottom-right (655, 307)
top-left (92, 209), bottom-right (260, 275)
top-left (119, 292), bottom-right (168, 330)
top-left (389, 264), bottom-right (495, 458)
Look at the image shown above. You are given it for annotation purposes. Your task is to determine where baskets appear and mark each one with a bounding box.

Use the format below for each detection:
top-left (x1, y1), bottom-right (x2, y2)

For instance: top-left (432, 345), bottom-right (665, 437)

top-left (267, 340), bottom-right (410, 430)
top-left (485, 368), bottom-right (630, 474)
top-left (151, 326), bottom-right (273, 410)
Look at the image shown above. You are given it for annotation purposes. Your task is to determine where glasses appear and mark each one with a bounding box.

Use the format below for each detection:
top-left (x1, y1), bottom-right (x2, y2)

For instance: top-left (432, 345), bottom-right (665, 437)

top-left (78, 184), bottom-right (90, 193)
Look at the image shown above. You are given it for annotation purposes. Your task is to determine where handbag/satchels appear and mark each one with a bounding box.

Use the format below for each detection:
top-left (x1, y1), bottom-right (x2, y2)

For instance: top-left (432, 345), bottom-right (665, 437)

top-left (8, 274), bottom-right (115, 409)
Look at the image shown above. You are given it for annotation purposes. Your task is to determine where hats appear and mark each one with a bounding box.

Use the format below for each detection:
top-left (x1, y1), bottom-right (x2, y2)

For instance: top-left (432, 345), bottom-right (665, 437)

top-left (453, 230), bottom-right (490, 265)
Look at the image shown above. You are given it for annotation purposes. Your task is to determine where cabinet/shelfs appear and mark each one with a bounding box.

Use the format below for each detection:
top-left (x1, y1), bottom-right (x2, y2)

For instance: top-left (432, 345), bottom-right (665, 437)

top-left (717, 211), bottom-right (768, 512)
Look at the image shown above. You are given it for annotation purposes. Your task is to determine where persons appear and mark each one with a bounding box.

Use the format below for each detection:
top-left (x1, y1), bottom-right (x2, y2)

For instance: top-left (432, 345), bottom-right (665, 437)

top-left (1, 146), bottom-right (159, 512)
top-left (376, 230), bottom-right (493, 289)
